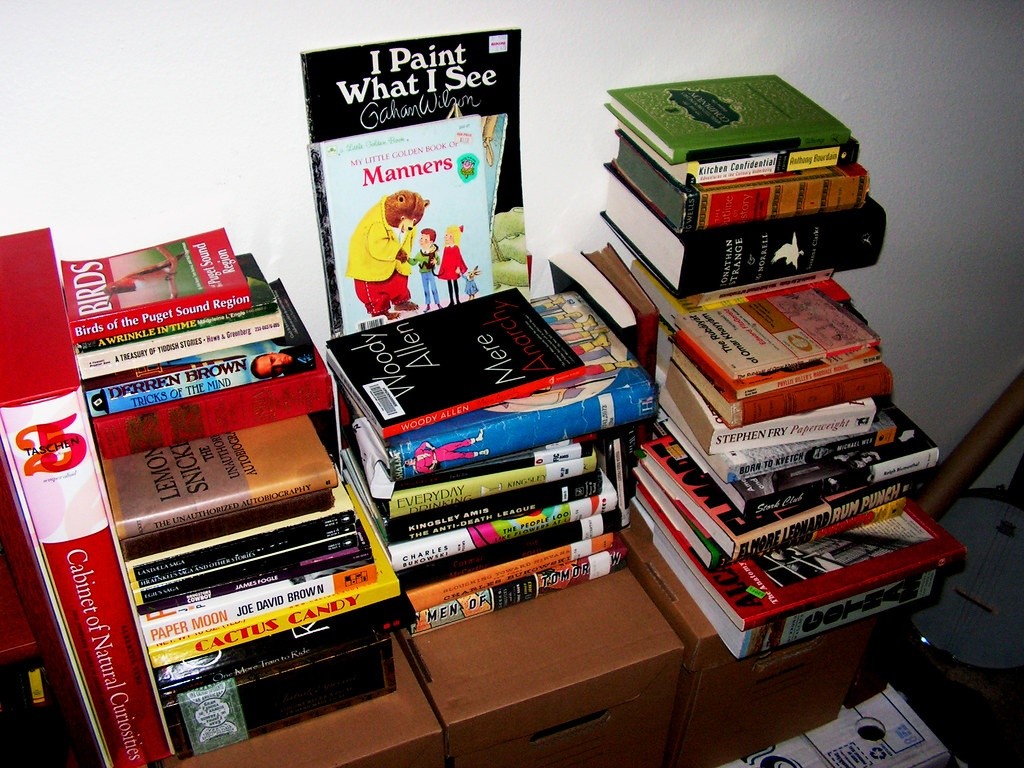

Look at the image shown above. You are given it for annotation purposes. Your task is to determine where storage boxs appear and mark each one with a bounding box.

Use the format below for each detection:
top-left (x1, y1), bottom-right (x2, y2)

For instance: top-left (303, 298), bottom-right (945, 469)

top-left (157, 497), bottom-right (949, 768)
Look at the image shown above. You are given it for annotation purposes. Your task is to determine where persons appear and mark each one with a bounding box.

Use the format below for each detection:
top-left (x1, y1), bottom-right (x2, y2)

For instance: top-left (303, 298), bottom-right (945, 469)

top-left (803, 446), bottom-right (834, 465)
top-left (849, 452), bottom-right (881, 469)
top-left (250, 344), bottom-right (317, 379)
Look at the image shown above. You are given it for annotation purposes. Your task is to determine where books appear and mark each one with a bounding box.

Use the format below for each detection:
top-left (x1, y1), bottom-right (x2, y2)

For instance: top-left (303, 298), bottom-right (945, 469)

top-left (300, 27), bottom-right (531, 340)
top-left (0, 77), bottom-right (967, 768)
